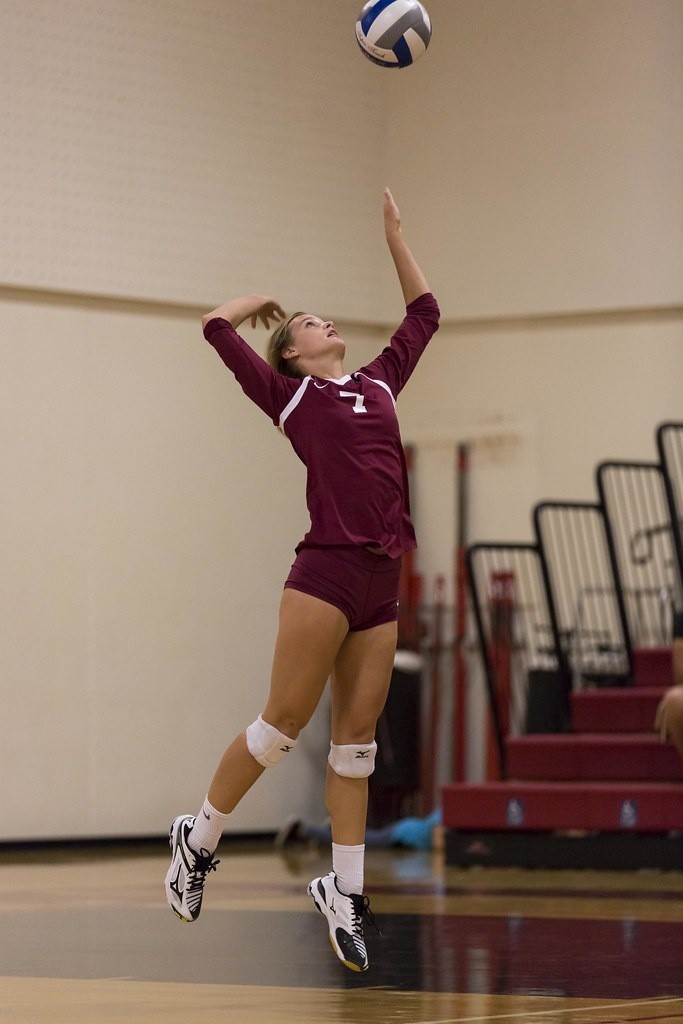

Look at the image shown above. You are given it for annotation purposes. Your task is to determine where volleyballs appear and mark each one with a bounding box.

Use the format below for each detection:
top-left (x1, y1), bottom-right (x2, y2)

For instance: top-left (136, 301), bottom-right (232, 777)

top-left (347, 0), bottom-right (436, 64)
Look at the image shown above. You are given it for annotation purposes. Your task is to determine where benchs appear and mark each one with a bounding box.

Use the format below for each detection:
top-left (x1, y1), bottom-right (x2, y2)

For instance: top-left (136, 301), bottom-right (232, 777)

top-left (441, 784), bottom-right (683, 870)
top-left (507, 734), bottom-right (683, 782)
top-left (635, 648), bottom-right (676, 685)
top-left (572, 686), bottom-right (670, 734)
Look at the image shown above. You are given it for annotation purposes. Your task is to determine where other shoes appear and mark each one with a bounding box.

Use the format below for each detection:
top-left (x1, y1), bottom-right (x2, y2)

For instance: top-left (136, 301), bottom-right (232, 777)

top-left (279, 819), bottom-right (302, 850)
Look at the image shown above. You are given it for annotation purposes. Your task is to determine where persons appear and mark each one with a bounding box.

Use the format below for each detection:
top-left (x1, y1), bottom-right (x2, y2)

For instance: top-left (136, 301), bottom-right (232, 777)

top-left (167, 189), bottom-right (440, 975)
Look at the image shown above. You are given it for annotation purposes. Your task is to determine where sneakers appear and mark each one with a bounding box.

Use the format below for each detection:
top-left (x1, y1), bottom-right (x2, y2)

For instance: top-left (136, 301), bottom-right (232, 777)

top-left (307, 871), bottom-right (373, 972)
top-left (165, 814), bottom-right (220, 922)
